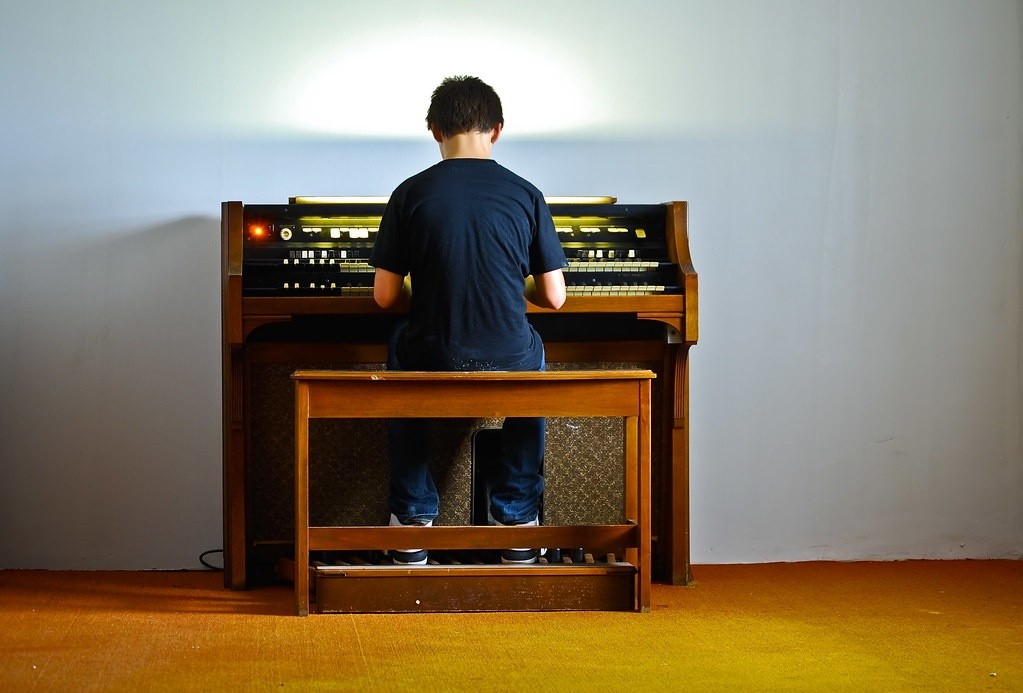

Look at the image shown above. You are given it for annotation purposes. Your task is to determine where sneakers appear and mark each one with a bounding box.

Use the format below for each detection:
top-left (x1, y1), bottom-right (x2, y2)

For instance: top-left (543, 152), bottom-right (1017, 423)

top-left (381, 511), bottom-right (434, 565)
top-left (493, 515), bottom-right (548, 564)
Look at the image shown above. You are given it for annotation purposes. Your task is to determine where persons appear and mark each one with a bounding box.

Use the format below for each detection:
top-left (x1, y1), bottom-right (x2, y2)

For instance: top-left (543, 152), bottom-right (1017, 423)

top-left (367, 75), bottom-right (569, 564)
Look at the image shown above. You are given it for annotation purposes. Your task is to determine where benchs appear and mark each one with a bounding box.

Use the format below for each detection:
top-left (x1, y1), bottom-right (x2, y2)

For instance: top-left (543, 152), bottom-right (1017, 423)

top-left (292, 368), bottom-right (658, 617)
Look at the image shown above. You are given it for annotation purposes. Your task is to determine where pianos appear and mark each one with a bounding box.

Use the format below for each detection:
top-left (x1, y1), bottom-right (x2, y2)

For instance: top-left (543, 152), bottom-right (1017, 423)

top-left (219, 193), bottom-right (700, 594)
